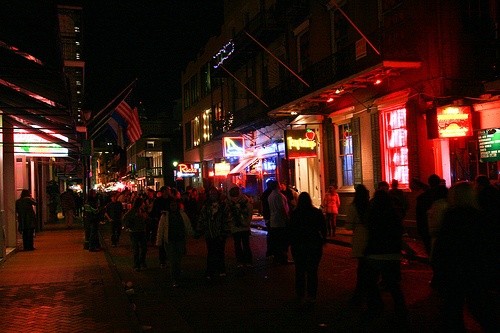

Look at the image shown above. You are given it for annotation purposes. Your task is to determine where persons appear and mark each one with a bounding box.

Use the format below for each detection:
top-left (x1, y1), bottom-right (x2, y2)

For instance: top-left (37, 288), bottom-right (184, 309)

top-left (285, 192), bottom-right (327, 305)
top-left (429, 181), bottom-right (500, 333)
top-left (322, 179), bottom-right (410, 293)
top-left (15, 176), bottom-right (300, 300)
top-left (356, 190), bottom-right (413, 330)
top-left (413, 175), bottom-right (500, 309)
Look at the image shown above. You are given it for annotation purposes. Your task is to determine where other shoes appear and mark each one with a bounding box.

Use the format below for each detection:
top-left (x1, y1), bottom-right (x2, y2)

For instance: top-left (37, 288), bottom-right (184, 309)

top-left (136, 267), bottom-right (140, 271)
top-left (297, 294), bottom-right (303, 299)
top-left (160, 264), bottom-right (163, 269)
top-left (307, 296), bottom-right (316, 302)
top-left (112, 244), bottom-right (115, 247)
top-left (143, 262), bottom-right (147, 268)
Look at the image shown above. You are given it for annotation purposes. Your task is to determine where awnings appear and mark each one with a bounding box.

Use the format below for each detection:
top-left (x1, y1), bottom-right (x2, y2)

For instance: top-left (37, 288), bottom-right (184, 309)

top-left (266, 60), bottom-right (422, 121)
top-left (215, 114), bottom-right (280, 139)
